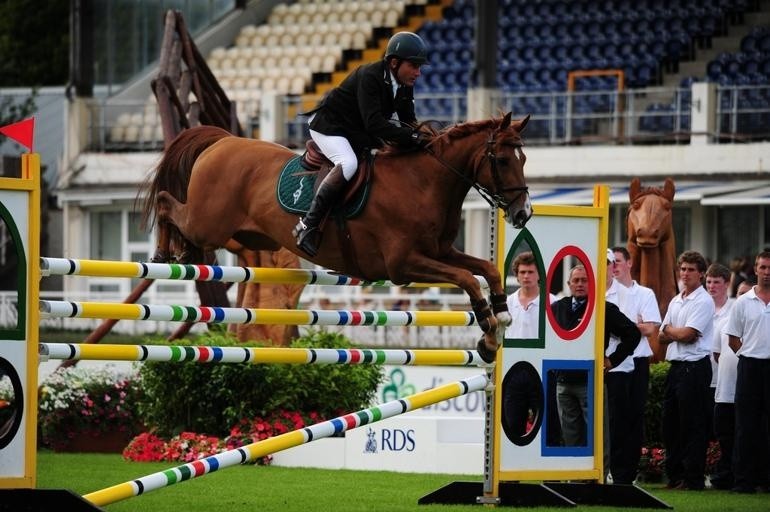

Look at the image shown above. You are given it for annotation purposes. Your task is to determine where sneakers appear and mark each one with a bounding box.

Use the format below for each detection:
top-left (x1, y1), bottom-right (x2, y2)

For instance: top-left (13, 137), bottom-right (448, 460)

top-left (659, 475), bottom-right (753, 493)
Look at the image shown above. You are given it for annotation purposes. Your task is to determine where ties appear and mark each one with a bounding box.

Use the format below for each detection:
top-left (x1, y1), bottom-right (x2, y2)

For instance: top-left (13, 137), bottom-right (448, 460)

top-left (573, 301), bottom-right (582, 311)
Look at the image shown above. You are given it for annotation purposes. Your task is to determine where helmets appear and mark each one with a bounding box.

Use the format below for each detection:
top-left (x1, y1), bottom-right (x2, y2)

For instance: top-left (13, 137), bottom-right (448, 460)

top-left (384, 31), bottom-right (431, 65)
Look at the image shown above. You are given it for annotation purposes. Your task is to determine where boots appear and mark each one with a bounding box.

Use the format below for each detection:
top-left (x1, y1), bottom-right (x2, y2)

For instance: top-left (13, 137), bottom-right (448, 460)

top-left (291, 164), bottom-right (349, 257)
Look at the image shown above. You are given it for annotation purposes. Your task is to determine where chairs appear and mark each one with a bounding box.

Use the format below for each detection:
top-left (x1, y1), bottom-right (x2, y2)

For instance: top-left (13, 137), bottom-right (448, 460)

top-left (102, 0), bottom-right (769, 151)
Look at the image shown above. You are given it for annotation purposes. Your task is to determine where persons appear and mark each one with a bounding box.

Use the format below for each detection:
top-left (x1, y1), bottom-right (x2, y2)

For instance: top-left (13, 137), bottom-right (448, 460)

top-left (603, 247), bottom-right (639, 501)
top-left (710, 280), bottom-right (754, 490)
top-left (605, 247), bottom-right (662, 484)
top-left (726, 256), bottom-right (751, 299)
top-left (503, 252), bottom-right (540, 485)
top-left (724, 252), bottom-right (770, 494)
top-left (660, 252), bottom-right (716, 491)
top-left (704, 262), bottom-right (736, 444)
top-left (546, 264), bottom-right (642, 484)
top-left (291, 32), bottom-right (429, 256)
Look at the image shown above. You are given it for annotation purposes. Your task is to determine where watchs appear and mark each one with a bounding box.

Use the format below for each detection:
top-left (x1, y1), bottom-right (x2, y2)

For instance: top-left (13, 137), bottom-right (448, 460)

top-left (662, 324), bottom-right (667, 331)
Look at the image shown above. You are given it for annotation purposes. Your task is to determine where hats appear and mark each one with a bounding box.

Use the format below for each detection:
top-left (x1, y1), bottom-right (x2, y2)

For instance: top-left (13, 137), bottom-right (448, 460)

top-left (607, 247), bottom-right (617, 268)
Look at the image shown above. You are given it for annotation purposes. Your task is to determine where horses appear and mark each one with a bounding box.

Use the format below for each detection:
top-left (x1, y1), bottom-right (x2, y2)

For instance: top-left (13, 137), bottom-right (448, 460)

top-left (625, 174), bottom-right (680, 364)
top-left (126, 108), bottom-right (535, 365)
